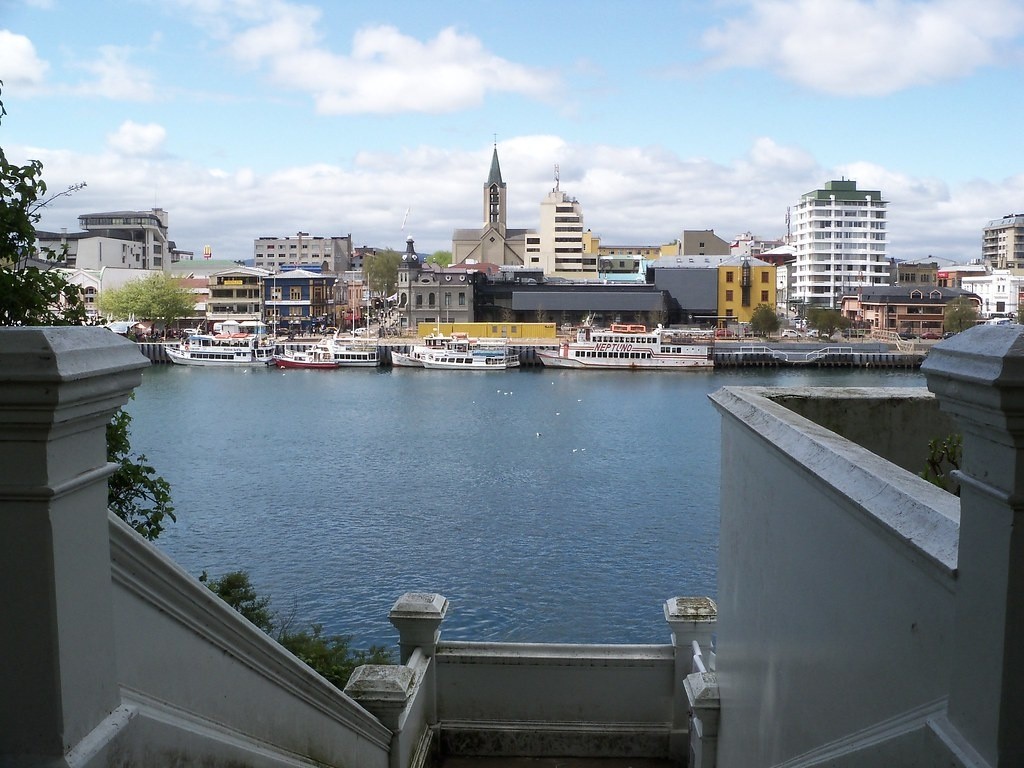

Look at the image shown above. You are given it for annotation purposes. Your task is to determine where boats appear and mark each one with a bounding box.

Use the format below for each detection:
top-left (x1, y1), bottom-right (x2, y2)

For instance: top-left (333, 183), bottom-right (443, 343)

top-left (272, 345), bottom-right (341, 370)
top-left (419, 347), bottom-right (507, 370)
top-left (165, 335), bottom-right (276, 368)
top-left (534, 323), bottom-right (715, 371)
top-left (390, 340), bottom-right (521, 368)
top-left (317, 337), bottom-right (381, 367)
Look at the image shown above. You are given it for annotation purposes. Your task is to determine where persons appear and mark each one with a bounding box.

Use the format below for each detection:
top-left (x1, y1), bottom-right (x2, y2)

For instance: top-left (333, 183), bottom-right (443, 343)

top-left (297, 325), bottom-right (320, 337)
top-left (134, 328), bottom-right (190, 346)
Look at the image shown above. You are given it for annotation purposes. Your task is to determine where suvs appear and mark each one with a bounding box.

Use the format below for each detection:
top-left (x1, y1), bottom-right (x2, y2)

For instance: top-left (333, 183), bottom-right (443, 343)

top-left (842, 327), bottom-right (866, 339)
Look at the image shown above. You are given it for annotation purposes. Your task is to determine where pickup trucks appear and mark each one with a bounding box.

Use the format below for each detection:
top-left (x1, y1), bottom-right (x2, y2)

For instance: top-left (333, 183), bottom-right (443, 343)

top-left (805, 329), bottom-right (829, 338)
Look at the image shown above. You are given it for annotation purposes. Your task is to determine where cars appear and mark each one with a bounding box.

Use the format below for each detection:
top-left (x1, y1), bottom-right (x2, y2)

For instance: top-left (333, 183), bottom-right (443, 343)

top-left (943, 332), bottom-right (955, 340)
top-left (920, 331), bottom-right (942, 340)
top-left (744, 330), bottom-right (766, 338)
top-left (350, 327), bottom-right (374, 337)
top-left (385, 327), bottom-right (398, 335)
top-left (715, 329), bottom-right (740, 338)
top-left (899, 332), bottom-right (916, 341)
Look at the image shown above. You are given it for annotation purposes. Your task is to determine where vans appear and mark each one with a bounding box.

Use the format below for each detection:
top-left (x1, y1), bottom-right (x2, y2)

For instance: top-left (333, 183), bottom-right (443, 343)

top-left (782, 329), bottom-right (802, 338)
